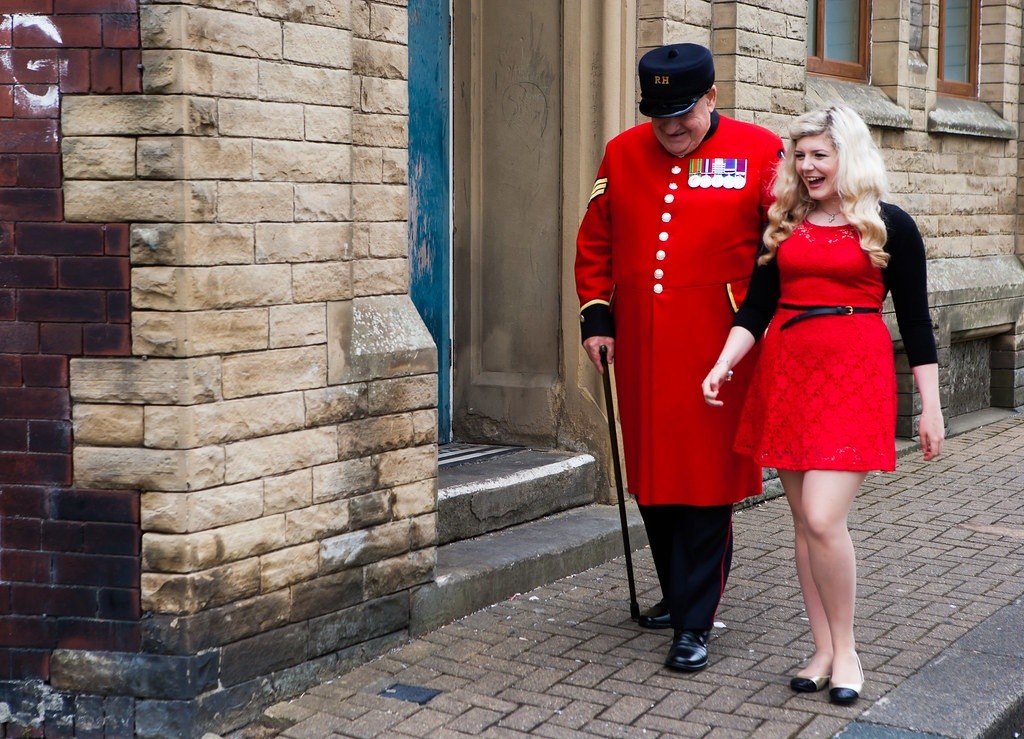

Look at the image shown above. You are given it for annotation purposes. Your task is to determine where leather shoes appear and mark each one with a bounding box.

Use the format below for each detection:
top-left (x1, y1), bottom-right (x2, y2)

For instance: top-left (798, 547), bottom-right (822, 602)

top-left (790, 669), bottom-right (830, 692)
top-left (638, 600), bottom-right (671, 629)
top-left (829, 651), bottom-right (864, 703)
top-left (665, 629), bottom-right (709, 671)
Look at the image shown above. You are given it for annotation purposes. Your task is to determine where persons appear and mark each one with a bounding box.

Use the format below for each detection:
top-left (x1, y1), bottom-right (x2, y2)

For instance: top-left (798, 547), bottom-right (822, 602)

top-left (574, 42), bottom-right (786, 673)
top-left (699, 102), bottom-right (947, 705)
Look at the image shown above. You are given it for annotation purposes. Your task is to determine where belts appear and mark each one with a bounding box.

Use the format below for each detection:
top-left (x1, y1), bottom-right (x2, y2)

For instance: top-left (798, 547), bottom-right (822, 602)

top-left (781, 303), bottom-right (879, 330)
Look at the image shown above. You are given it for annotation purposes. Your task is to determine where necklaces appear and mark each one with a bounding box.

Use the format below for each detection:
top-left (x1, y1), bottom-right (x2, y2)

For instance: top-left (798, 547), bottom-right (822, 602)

top-left (821, 206), bottom-right (842, 223)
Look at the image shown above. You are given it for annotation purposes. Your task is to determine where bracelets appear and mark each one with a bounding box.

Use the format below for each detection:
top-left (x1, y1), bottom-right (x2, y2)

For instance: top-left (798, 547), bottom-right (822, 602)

top-left (717, 358), bottom-right (733, 381)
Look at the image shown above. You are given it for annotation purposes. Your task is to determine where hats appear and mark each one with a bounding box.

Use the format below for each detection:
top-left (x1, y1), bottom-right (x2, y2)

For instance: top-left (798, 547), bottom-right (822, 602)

top-left (639, 42), bottom-right (714, 118)
